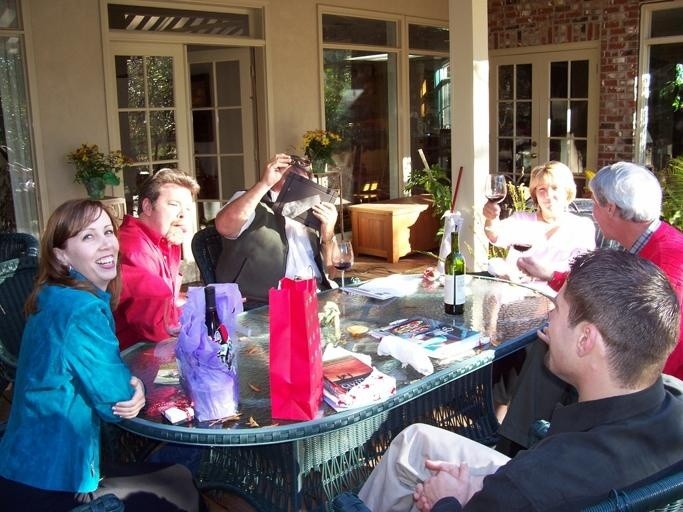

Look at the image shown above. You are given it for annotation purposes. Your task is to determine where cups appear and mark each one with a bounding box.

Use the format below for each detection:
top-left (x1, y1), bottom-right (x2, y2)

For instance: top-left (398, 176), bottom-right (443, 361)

top-left (164, 298), bottom-right (191, 339)
top-left (331, 239), bottom-right (355, 298)
top-left (511, 210), bottom-right (538, 283)
top-left (485, 172), bottom-right (507, 231)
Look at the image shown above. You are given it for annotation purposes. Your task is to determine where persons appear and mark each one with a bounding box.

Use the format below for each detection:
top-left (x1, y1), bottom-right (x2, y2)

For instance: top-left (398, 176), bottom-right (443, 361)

top-left (483, 162), bottom-right (595, 298)
top-left (216, 153), bottom-right (338, 309)
top-left (1, 199), bottom-right (199, 512)
top-left (589, 160), bottom-right (682, 378)
top-left (357, 251), bottom-right (682, 510)
top-left (103, 167), bottom-right (198, 351)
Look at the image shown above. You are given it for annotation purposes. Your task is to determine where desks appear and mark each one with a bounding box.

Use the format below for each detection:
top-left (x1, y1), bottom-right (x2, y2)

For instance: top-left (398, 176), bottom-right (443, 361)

top-left (350, 193), bottom-right (442, 264)
top-left (108, 271), bottom-right (557, 512)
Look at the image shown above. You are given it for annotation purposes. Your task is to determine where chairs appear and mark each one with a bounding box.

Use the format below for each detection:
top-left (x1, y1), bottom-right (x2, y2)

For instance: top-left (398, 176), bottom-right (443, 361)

top-left (568, 197), bottom-right (613, 252)
top-left (580, 371), bottom-right (682, 512)
top-left (0, 257), bottom-right (43, 384)
top-left (192, 227), bottom-right (222, 312)
top-left (2, 233), bottom-right (40, 260)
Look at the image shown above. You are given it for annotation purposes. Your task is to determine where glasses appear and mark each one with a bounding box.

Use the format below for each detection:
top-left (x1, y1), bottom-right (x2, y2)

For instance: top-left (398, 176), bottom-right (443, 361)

top-left (289, 156), bottom-right (311, 168)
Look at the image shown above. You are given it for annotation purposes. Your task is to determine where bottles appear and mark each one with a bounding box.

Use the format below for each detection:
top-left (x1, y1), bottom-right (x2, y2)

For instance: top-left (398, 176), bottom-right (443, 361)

top-left (444, 232), bottom-right (465, 315)
top-left (201, 286), bottom-right (221, 339)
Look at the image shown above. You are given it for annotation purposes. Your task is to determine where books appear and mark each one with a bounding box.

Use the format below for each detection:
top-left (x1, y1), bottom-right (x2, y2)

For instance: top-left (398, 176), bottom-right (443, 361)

top-left (339, 281), bottom-right (410, 300)
top-left (373, 315), bottom-right (488, 359)
top-left (322, 355), bottom-right (395, 401)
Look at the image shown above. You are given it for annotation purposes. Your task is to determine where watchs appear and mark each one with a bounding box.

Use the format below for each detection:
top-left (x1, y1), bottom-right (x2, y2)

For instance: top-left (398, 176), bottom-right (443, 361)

top-left (321, 236), bottom-right (337, 244)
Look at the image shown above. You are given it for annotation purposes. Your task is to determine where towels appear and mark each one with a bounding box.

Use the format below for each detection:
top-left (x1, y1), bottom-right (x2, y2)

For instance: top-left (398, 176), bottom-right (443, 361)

top-left (376, 334), bottom-right (435, 378)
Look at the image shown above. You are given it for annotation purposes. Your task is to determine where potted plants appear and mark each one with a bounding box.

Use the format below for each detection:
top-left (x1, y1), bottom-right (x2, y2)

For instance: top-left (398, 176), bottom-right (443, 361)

top-left (66, 143), bottom-right (134, 228)
top-left (302, 130), bottom-right (345, 188)
